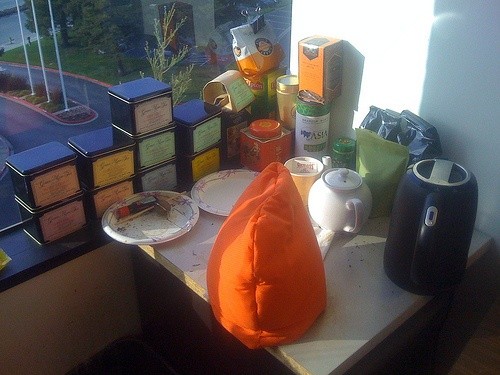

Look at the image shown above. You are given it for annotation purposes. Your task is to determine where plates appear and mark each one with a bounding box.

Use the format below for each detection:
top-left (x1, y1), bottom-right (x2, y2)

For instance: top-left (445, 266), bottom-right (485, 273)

top-left (191, 169), bottom-right (263, 217)
top-left (101, 191), bottom-right (200, 245)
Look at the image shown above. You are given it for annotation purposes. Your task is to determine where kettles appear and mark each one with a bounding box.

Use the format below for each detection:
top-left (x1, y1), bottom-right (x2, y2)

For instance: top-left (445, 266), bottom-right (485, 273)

top-left (383, 157), bottom-right (478, 296)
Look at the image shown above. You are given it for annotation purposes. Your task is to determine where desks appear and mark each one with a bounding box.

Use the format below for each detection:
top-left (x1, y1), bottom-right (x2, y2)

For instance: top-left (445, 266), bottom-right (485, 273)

top-left (138, 216), bottom-right (492, 375)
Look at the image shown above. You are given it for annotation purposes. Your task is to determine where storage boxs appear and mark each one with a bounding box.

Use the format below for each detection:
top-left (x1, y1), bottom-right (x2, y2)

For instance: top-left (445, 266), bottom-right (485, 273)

top-left (4, 36), bottom-right (344, 248)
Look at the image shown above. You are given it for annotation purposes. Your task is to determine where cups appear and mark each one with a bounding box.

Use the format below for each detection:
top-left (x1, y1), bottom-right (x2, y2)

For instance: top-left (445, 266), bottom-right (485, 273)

top-left (284, 156), bottom-right (323, 206)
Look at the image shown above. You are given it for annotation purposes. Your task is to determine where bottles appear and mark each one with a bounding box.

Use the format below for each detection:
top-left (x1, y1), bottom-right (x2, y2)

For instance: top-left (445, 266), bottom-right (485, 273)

top-left (332, 138), bottom-right (359, 170)
top-left (237, 119), bottom-right (297, 169)
top-left (295, 96), bottom-right (333, 160)
top-left (275, 75), bottom-right (302, 130)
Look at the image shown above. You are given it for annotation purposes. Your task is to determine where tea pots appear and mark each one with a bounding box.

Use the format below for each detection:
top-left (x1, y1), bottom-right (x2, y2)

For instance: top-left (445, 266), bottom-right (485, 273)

top-left (308, 156), bottom-right (373, 234)
